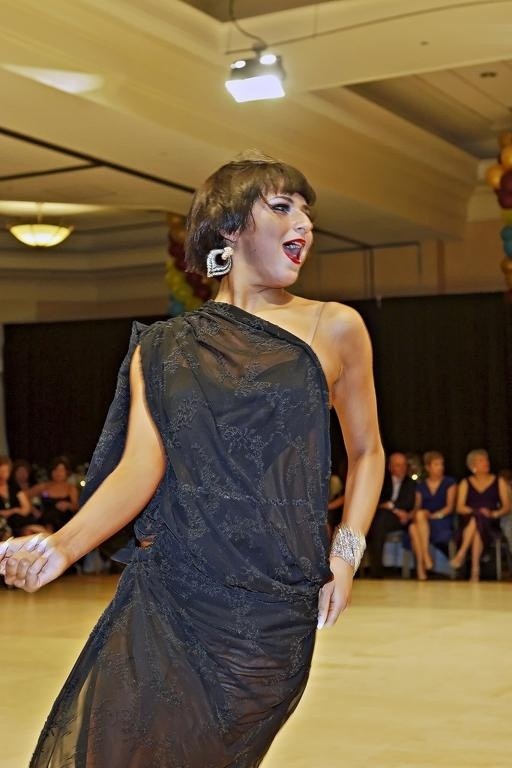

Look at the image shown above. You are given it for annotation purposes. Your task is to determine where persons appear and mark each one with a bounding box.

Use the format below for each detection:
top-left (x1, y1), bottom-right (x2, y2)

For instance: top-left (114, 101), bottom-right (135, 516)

top-left (327, 450), bottom-right (512, 583)
top-left (1, 452), bottom-right (82, 542)
top-left (0, 149), bottom-right (387, 768)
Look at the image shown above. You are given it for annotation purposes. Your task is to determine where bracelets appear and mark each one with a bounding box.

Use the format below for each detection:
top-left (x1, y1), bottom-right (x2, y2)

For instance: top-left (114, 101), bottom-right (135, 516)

top-left (326, 523), bottom-right (368, 576)
